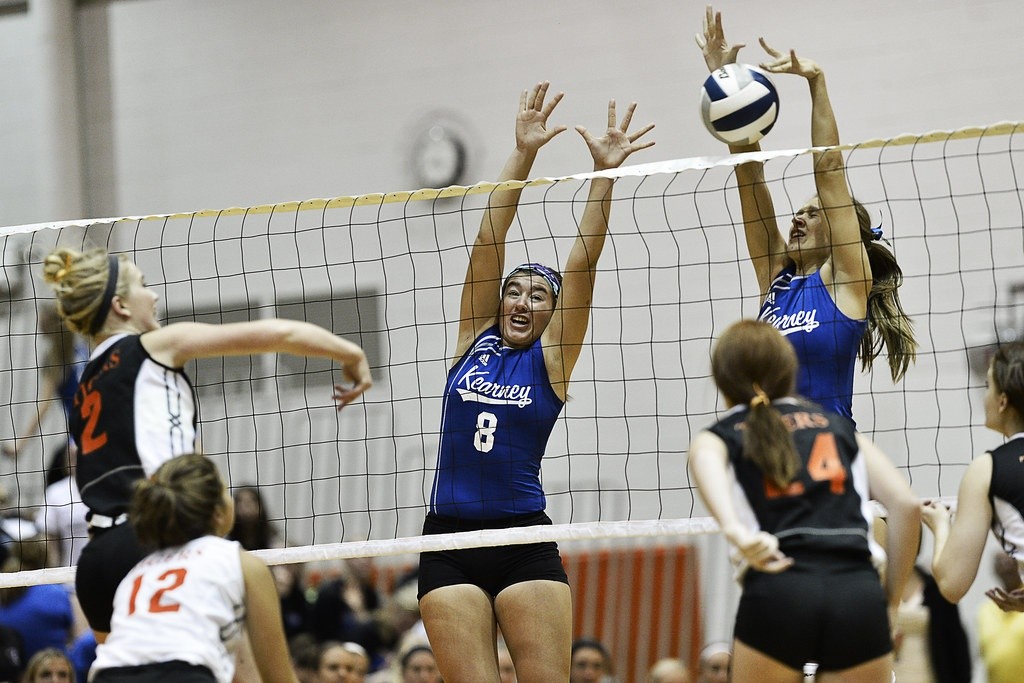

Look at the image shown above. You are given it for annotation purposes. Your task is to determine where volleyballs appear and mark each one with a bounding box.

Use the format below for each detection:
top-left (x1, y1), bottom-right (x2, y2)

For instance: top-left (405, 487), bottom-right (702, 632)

top-left (699, 59), bottom-right (783, 151)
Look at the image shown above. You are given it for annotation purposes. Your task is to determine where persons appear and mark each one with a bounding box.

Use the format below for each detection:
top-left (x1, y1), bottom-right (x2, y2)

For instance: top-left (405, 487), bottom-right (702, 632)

top-left (84, 449), bottom-right (302, 683)
top-left (872, 336), bottom-right (1024, 683)
top-left (413, 77), bottom-right (658, 683)
top-left (692, 2), bottom-right (920, 427)
top-left (491, 636), bottom-right (735, 683)
top-left (41, 242), bottom-right (374, 646)
top-left (691, 320), bottom-right (924, 683)
top-left (0, 432), bottom-right (442, 683)
